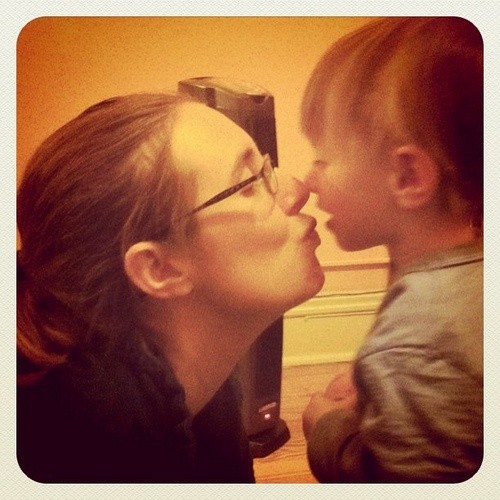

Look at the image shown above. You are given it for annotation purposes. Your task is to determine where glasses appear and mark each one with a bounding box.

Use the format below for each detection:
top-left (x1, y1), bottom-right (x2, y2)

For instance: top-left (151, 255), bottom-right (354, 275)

top-left (151, 153), bottom-right (278, 240)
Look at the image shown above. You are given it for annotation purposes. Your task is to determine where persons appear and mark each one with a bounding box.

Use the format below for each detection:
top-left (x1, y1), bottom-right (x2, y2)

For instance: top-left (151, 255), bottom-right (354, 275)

top-left (17, 93), bottom-right (325, 484)
top-left (300, 17), bottom-right (483, 483)
